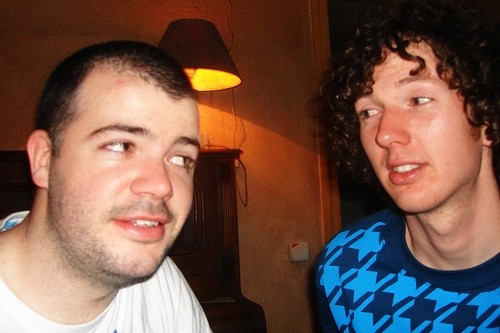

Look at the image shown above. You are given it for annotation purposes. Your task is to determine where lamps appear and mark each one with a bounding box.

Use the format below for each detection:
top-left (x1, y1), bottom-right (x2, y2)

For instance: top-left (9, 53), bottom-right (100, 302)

top-left (156, 18), bottom-right (242, 94)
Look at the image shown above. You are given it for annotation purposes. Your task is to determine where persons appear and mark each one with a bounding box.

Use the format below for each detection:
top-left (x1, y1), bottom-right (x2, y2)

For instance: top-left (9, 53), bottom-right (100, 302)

top-left (0, 41), bottom-right (213, 333)
top-left (308, 0), bottom-right (499, 333)
